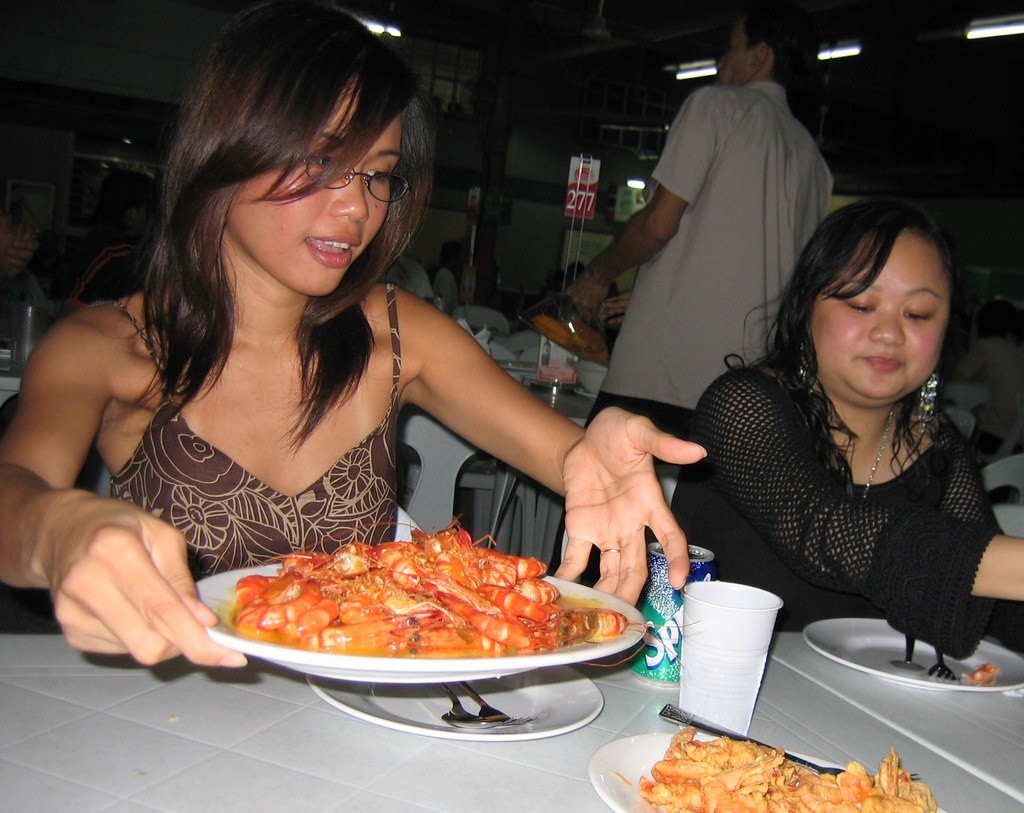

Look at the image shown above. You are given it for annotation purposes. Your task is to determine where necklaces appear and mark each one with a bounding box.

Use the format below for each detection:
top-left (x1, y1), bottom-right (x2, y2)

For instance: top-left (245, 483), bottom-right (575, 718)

top-left (856, 409), bottom-right (894, 501)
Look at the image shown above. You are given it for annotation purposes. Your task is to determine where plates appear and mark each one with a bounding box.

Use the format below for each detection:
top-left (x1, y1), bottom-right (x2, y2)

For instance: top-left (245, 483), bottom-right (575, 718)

top-left (589, 733), bottom-right (862, 813)
top-left (573, 388), bottom-right (597, 402)
top-left (0, 338), bottom-right (11, 356)
top-left (803, 617), bottom-right (1024, 692)
top-left (195, 563), bottom-right (646, 683)
top-left (299, 666), bottom-right (604, 742)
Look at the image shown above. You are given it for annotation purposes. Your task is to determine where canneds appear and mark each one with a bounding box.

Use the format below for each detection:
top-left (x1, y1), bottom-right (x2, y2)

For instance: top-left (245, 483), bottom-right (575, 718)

top-left (625, 543), bottom-right (715, 689)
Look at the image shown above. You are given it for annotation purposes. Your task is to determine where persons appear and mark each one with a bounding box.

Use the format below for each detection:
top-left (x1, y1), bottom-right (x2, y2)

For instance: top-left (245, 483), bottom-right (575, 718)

top-left (546, 0), bottom-right (833, 609)
top-left (0, 0), bottom-right (710, 667)
top-left (67, 168), bottom-right (585, 315)
top-left (670, 195), bottom-right (1024, 660)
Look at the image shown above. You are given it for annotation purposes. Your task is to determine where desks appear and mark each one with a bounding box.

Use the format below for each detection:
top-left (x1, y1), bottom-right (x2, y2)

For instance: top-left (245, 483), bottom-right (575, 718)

top-left (459, 382), bottom-right (591, 570)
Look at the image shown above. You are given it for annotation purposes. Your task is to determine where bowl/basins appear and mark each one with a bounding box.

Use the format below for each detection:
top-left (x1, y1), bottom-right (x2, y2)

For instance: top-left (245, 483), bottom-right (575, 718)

top-left (572, 364), bottom-right (608, 395)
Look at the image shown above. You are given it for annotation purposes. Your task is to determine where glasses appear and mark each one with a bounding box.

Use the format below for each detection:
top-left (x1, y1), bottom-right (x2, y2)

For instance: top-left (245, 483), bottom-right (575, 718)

top-left (297, 154), bottom-right (413, 201)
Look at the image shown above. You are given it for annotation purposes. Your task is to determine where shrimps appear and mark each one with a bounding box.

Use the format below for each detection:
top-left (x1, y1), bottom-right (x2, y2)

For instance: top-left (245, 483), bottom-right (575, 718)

top-left (231, 506), bottom-right (630, 653)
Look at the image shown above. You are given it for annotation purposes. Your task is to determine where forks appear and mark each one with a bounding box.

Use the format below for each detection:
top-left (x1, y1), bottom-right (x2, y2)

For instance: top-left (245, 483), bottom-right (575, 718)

top-left (659, 703), bottom-right (921, 782)
top-left (456, 682), bottom-right (534, 726)
top-left (928, 645), bottom-right (961, 681)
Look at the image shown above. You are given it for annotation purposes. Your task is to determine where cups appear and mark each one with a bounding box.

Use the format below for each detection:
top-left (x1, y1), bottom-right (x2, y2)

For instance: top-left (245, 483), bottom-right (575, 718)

top-left (677, 581), bottom-right (784, 736)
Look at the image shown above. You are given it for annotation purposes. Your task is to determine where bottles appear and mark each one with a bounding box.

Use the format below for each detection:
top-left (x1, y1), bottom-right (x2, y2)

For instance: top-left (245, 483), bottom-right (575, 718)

top-left (11, 304), bottom-right (42, 377)
top-left (541, 338), bottom-right (551, 365)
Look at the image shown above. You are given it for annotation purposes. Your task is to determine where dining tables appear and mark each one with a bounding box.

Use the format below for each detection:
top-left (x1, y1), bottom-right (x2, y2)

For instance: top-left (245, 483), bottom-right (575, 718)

top-left (0, 632), bottom-right (1024, 813)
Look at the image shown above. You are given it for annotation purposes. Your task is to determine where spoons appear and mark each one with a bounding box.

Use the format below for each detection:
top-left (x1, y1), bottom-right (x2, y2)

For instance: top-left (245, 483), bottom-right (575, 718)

top-left (439, 683), bottom-right (501, 730)
top-left (889, 634), bottom-right (925, 671)
